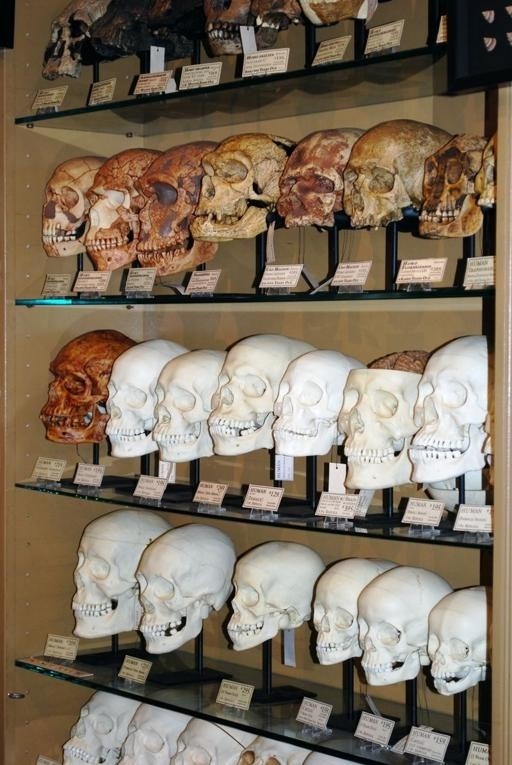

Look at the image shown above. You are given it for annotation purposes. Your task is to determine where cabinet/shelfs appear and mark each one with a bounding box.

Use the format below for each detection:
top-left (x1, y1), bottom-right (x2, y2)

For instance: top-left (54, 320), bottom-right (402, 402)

top-left (4, 22), bottom-right (511, 764)
